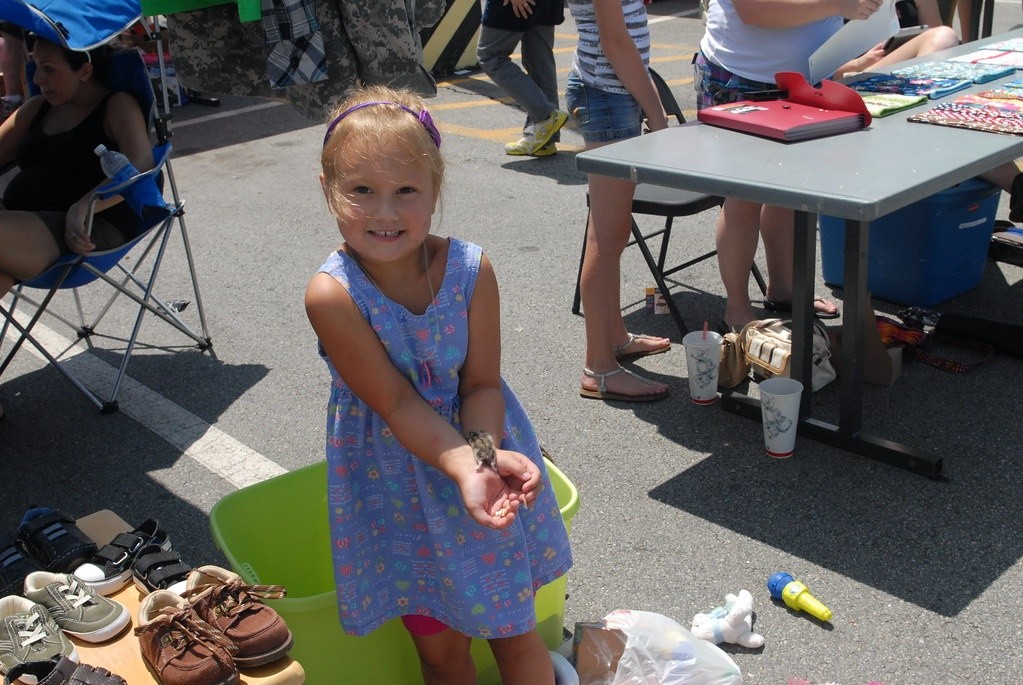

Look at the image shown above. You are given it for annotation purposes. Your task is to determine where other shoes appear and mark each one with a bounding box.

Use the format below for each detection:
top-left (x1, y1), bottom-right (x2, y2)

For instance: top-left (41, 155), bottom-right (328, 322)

top-left (1009, 172), bottom-right (1023, 222)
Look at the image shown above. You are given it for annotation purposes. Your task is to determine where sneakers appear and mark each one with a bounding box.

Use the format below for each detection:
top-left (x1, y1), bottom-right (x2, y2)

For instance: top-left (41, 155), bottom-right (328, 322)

top-left (135, 562), bottom-right (294, 685)
top-left (73, 516), bottom-right (173, 596)
top-left (1, 507), bottom-right (99, 600)
top-left (25, 571), bottom-right (131, 643)
top-left (504, 134), bottom-right (557, 156)
top-left (526, 108), bottom-right (569, 155)
top-left (0, 593), bottom-right (80, 685)
top-left (132, 543), bottom-right (193, 599)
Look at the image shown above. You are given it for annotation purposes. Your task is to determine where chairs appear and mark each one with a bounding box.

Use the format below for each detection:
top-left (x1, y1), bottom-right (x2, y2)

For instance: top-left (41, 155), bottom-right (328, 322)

top-left (571, 67), bottom-right (767, 340)
top-left (0, 0), bottom-right (208, 420)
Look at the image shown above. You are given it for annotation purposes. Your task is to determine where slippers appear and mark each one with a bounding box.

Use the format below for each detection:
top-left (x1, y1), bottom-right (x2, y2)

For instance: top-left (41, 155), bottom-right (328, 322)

top-left (763, 295), bottom-right (840, 317)
top-left (719, 320), bottom-right (733, 334)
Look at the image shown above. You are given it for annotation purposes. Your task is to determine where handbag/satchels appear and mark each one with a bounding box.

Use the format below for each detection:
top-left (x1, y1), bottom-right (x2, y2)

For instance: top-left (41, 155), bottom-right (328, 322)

top-left (719, 319), bottom-right (762, 387)
top-left (744, 318), bottom-right (837, 392)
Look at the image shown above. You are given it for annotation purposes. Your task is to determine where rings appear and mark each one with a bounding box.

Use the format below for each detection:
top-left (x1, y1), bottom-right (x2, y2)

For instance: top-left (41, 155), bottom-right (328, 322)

top-left (524, 3), bottom-right (528, 6)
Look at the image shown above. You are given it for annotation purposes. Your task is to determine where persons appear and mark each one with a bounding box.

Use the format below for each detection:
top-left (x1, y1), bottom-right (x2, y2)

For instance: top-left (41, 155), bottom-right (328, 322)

top-left (565, 2), bottom-right (672, 400)
top-left (476, 0), bottom-right (569, 157)
top-left (0, 36), bottom-right (166, 300)
top-left (305, 85), bottom-right (574, 685)
top-left (691, 0), bottom-right (1023, 340)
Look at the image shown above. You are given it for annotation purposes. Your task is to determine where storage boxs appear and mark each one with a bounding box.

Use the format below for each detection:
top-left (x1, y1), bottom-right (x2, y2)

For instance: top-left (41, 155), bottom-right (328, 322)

top-left (211, 436), bottom-right (576, 685)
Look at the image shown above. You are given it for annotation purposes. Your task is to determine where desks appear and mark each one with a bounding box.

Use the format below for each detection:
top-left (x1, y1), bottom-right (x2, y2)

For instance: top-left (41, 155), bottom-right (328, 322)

top-left (574, 29), bottom-right (1023, 480)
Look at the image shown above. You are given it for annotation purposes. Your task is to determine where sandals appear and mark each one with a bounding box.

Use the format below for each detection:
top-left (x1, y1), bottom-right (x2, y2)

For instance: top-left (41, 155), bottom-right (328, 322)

top-left (579, 361), bottom-right (669, 401)
top-left (613, 332), bottom-right (671, 357)
top-left (3, 652), bottom-right (127, 685)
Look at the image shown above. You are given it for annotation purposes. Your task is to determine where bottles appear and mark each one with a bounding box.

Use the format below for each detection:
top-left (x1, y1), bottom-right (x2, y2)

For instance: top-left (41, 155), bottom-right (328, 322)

top-left (93, 144), bottom-right (141, 184)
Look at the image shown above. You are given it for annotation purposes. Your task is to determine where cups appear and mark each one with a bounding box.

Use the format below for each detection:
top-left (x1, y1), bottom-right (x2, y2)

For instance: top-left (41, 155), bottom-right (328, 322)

top-left (757, 378), bottom-right (805, 459)
top-left (681, 330), bottom-right (726, 405)
